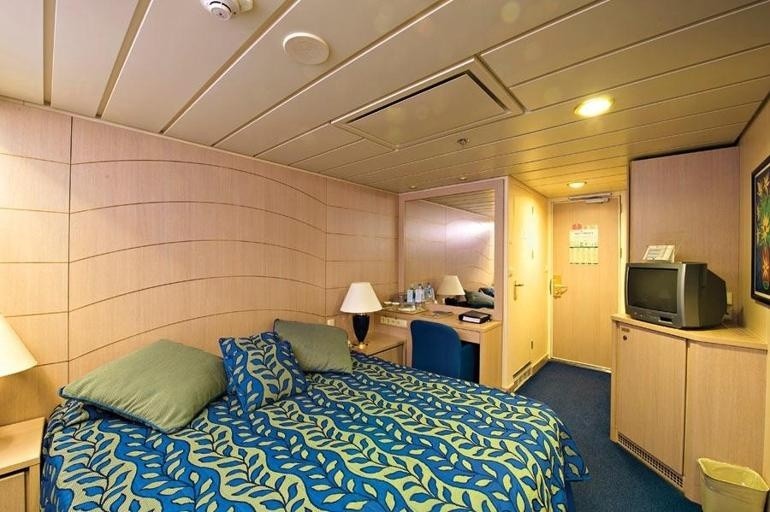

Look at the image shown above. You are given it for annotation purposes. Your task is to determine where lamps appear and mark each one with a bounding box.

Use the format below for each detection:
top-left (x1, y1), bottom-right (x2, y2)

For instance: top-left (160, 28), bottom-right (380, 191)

top-left (340, 282), bottom-right (382, 349)
top-left (437, 274), bottom-right (465, 305)
top-left (0, 313), bottom-right (37, 378)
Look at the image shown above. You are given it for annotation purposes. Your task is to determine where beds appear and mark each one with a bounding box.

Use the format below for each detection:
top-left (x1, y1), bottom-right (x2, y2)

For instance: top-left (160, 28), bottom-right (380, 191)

top-left (40, 348), bottom-right (590, 512)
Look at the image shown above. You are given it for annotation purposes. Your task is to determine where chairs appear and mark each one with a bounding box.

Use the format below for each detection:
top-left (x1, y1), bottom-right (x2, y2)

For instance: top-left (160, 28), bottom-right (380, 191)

top-left (411, 320), bottom-right (475, 381)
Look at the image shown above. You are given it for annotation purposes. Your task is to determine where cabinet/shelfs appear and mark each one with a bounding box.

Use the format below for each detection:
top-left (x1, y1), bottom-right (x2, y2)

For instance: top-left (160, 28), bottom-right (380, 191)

top-left (609, 312), bottom-right (770, 505)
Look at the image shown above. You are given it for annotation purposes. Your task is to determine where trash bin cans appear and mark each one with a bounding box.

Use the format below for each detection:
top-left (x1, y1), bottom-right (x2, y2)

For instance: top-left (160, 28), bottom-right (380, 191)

top-left (697, 457), bottom-right (770, 512)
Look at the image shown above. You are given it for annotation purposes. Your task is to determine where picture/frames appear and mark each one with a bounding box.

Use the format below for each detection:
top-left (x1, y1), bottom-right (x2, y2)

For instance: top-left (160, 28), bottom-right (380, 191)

top-left (751, 155), bottom-right (770, 305)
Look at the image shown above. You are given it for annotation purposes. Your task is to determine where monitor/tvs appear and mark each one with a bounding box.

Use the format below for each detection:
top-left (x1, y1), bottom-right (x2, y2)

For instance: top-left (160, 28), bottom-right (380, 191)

top-left (623, 261), bottom-right (728, 328)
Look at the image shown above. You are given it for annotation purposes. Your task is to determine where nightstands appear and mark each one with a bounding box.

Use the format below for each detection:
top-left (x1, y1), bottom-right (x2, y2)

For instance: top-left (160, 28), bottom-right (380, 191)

top-left (349, 331), bottom-right (407, 365)
top-left (0, 417), bottom-right (45, 512)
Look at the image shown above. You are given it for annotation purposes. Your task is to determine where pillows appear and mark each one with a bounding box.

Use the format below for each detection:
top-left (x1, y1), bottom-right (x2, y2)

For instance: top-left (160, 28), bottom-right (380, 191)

top-left (478, 288), bottom-right (494, 297)
top-left (464, 289), bottom-right (493, 307)
top-left (273, 318), bottom-right (355, 377)
top-left (219, 331), bottom-right (280, 395)
top-left (58, 340), bottom-right (227, 436)
top-left (225, 341), bottom-right (306, 413)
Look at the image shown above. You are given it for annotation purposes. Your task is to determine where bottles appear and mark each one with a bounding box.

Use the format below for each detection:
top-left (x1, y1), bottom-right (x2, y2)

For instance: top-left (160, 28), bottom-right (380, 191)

top-left (383, 282), bottom-right (435, 311)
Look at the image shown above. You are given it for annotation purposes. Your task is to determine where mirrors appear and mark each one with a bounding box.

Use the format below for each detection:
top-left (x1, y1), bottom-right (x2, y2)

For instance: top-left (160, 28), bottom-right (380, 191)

top-left (398, 176), bottom-right (503, 320)
